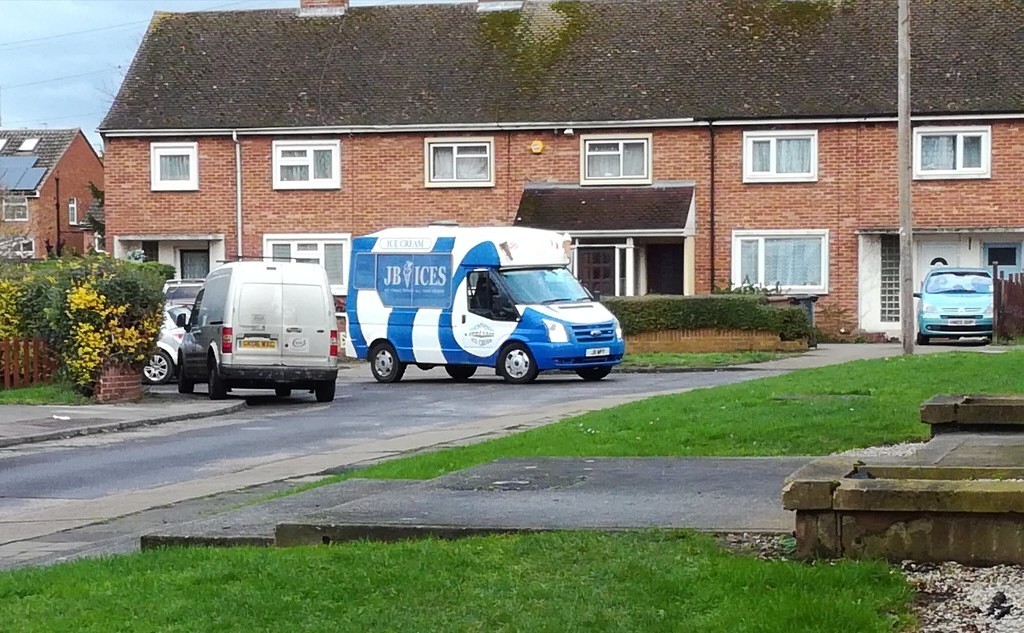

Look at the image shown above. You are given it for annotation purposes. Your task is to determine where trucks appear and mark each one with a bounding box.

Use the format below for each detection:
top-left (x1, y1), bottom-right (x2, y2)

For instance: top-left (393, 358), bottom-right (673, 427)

top-left (172, 257), bottom-right (338, 402)
top-left (344, 227), bottom-right (626, 385)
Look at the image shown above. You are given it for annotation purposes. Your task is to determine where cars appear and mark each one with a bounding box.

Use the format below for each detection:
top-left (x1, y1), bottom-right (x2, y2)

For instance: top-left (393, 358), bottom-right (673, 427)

top-left (912, 266), bottom-right (994, 345)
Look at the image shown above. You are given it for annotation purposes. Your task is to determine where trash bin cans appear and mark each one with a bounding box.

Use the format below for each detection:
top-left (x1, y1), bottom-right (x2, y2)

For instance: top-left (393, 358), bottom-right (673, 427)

top-left (788, 294), bottom-right (819, 328)
top-left (335, 312), bottom-right (346, 355)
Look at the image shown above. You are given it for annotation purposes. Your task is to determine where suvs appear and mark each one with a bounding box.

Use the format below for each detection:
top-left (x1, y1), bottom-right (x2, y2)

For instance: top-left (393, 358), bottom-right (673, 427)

top-left (141, 277), bottom-right (206, 385)
